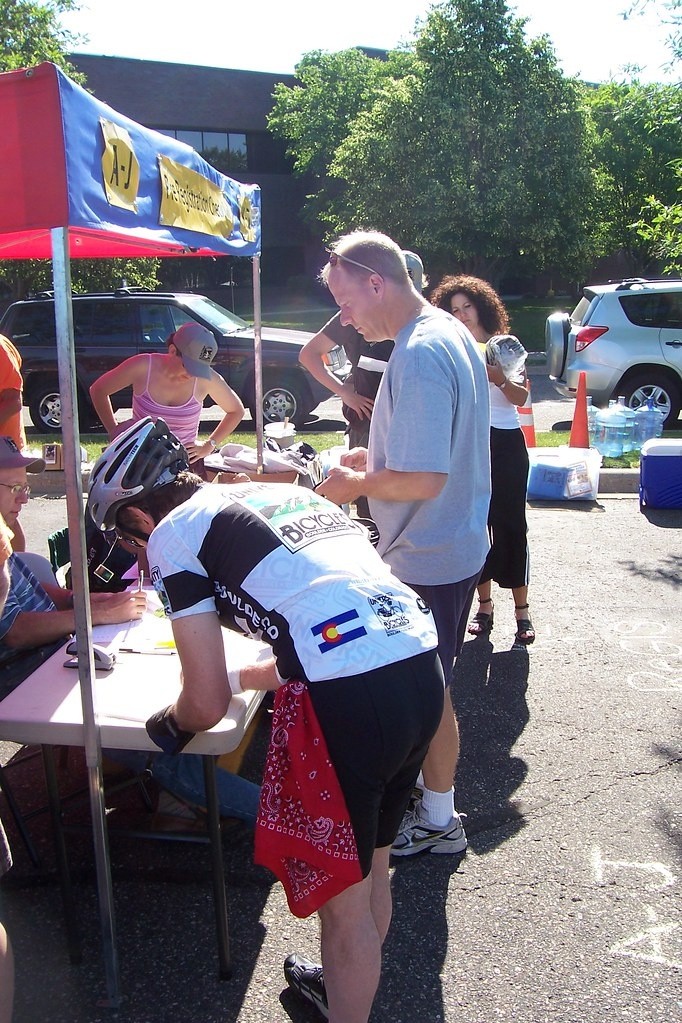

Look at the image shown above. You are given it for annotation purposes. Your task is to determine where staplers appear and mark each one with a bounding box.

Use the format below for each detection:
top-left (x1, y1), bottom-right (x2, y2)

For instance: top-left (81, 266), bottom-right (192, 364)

top-left (63, 641), bottom-right (116, 671)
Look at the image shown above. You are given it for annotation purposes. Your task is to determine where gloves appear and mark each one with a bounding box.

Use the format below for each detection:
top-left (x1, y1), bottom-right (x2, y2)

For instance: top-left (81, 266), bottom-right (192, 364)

top-left (146, 705), bottom-right (196, 757)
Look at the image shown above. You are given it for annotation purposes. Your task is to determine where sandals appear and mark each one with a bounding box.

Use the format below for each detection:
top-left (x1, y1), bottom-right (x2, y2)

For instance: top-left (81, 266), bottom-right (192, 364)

top-left (515, 603), bottom-right (534, 642)
top-left (468, 597), bottom-right (494, 633)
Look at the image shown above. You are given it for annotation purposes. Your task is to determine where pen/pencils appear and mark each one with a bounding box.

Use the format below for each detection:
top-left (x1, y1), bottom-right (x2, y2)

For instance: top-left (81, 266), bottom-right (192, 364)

top-left (138, 569), bottom-right (143, 592)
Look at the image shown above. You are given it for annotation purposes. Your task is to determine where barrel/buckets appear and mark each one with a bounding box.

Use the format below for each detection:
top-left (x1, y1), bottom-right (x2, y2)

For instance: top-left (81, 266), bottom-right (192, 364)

top-left (264, 422), bottom-right (296, 449)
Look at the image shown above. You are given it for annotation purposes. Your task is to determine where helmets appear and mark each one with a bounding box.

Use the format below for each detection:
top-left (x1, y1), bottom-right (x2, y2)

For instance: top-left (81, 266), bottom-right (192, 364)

top-left (88, 416), bottom-right (190, 530)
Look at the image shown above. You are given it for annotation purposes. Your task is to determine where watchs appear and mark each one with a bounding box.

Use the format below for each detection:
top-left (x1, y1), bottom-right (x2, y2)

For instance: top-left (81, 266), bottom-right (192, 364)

top-left (494, 375), bottom-right (506, 389)
top-left (205, 439), bottom-right (217, 450)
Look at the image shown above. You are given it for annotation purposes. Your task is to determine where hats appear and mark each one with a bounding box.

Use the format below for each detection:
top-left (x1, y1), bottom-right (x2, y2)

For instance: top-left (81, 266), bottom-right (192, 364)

top-left (0, 435), bottom-right (46, 474)
top-left (402, 250), bottom-right (423, 290)
top-left (173, 322), bottom-right (218, 380)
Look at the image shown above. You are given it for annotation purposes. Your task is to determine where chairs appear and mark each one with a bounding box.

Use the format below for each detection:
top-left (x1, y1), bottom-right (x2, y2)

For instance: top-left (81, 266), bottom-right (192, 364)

top-left (13, 553), bottom-right (59, 589)
top-left (48, 526), bottom-right (82, 587)
top-left (0, 747), bottom-right (157, 869)
top-left (147, 309), bottom-right (168, 342)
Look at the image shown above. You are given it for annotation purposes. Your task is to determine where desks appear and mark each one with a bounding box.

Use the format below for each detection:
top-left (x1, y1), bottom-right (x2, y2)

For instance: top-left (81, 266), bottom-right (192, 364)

top-left (0, 580), bottom-right (272, 982)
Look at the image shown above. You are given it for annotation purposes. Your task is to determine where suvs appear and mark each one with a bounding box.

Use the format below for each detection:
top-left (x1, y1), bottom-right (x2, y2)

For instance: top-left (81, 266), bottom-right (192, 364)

top-left (544, 277), bottom-right (682, 428)
top-left (0, 285), bottom-right (353, 431)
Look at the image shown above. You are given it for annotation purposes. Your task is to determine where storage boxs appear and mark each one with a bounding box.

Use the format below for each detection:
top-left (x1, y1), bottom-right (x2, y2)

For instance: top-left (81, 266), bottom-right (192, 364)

top-left (527, 448), bottom-right (602, 500)
top-left (42, 443), bottom-right (64, 471)
top-left (640, 437), bottom-right (682, 509)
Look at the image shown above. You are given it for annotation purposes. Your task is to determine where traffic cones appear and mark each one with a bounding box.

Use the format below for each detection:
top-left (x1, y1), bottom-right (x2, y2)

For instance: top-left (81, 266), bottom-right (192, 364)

top-left (568, 372), bottom-right (591, 449)
top-left (517, 378), bottom-right (537, 448)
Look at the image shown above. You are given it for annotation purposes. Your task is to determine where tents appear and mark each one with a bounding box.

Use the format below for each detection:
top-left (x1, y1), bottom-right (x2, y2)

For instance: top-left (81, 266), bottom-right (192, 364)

top-left (0, 61), bottom-right (268, 1010)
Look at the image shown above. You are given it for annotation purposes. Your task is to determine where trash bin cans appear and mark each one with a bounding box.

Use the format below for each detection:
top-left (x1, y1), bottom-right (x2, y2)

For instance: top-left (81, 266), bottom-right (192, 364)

top-left (264, 422), bottom-right (295, 449)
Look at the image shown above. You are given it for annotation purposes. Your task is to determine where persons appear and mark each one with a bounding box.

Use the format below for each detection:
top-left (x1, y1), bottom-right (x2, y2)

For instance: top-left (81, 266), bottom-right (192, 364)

top-left (297, 250), bottom-right (426, 518)
top-left (87, 412), bottom-right (447, 1023)
top-left (89, 322), bottom-right (246, 486)
top-left (0, 435), bottom-right (263, 825)
top-left (0, 332), bottom-right (26, 553)
top-left (312, 230), bottom-right (495, 862)
top-left (430, 276), bottom-right (538, 646)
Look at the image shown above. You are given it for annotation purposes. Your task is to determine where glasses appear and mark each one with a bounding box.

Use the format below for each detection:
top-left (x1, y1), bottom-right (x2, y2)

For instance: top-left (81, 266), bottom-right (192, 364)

top-left (329, 251), bottom-right (378, 274)
top-left (116, 529), bottom-right (144, 548)
top-left (174, 344), bottom-right (185, 368)
top-left (0, 483), bottom-right (32, 495)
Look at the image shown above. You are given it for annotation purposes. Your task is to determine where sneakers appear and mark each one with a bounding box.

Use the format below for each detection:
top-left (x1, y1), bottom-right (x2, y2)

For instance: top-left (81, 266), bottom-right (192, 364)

top-left (409, 769), bottom-right (424, 807)
top-left (284, 952), bottom-right (329, 1017)
top-left (388, 799), bottom-right (470, 857)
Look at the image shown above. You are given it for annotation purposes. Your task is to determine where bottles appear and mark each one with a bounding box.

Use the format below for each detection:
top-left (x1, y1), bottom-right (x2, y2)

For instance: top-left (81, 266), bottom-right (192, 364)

top-left (586, 395), bottom-right (664, 458)
